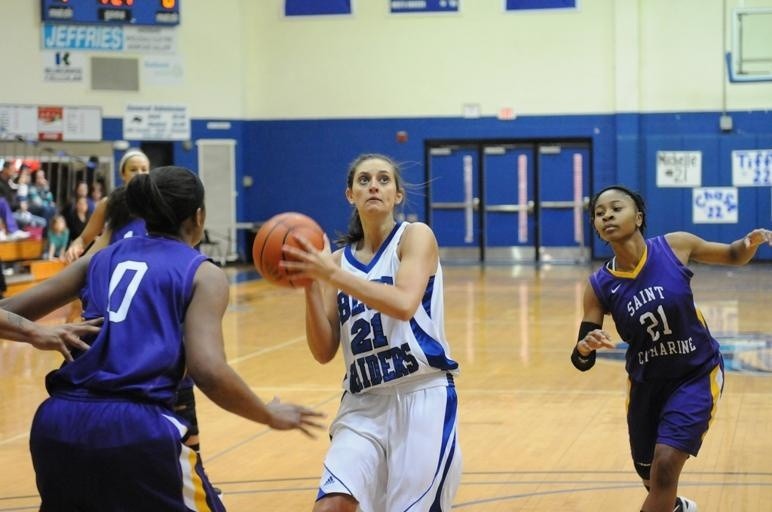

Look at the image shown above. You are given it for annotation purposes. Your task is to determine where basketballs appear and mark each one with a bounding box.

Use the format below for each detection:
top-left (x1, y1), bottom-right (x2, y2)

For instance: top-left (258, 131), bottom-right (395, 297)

top-left (253, 212), bottom-right (325, 287)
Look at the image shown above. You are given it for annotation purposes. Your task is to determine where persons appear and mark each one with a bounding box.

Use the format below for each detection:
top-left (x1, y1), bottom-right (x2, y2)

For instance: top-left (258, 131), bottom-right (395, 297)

top-left (62, 150), bottom-right (152, 265)
top-left (1, 164), bottom-right (328, 511)
top-left (569, 184), bottom-right (771, 511)
top-left (98, 184), bottom-right (233, 511)
top-left (273, 149), bottom-right (464, 511)
top-left (1, 159), bottom-right (108, 267)
top-left (0, 306), bottom-right (106, 363)
